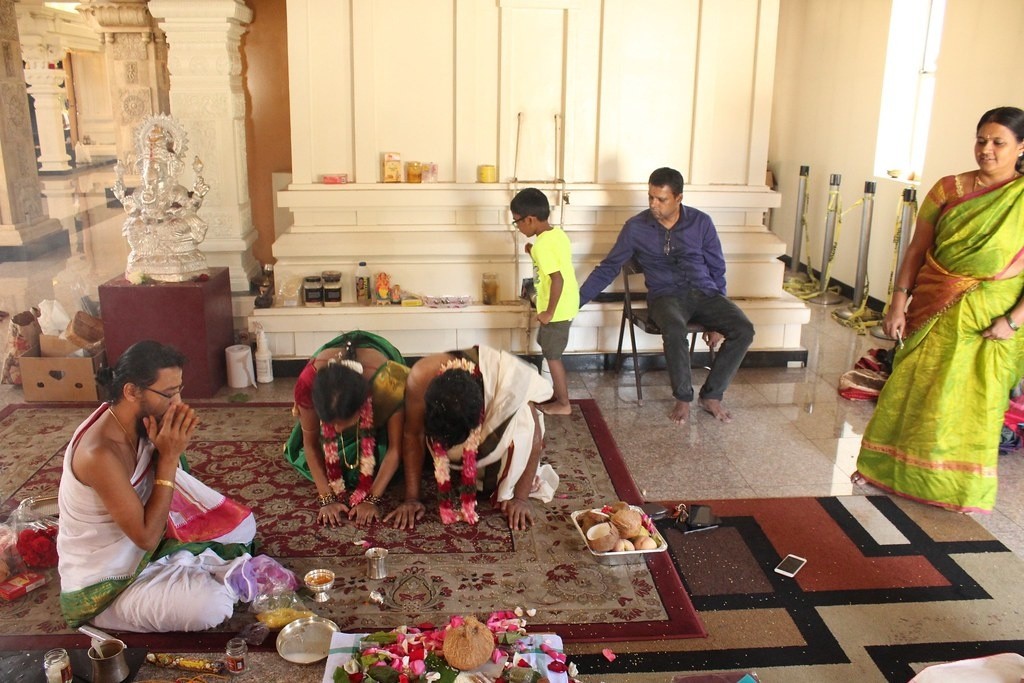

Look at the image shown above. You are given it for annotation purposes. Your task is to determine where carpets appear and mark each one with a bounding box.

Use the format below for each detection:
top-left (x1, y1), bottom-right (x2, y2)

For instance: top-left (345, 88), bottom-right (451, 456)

top-left (0, 398), bottom-right (709, 652)
top-left (563, 493), bottom-right (1023, 682)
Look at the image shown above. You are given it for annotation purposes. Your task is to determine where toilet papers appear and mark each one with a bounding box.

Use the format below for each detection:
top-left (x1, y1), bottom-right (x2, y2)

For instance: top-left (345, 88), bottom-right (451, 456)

top-left (225, 345), bottom-right (259, 389)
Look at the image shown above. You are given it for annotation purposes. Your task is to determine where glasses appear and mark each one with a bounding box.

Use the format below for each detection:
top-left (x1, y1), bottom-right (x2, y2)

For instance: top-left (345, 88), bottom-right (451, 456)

top-left (663, 230), bottom-right (671, 255)
top-left (514, 213), bottom-right (535, 228)
top-left (131, 380), bottom-right (187, 406)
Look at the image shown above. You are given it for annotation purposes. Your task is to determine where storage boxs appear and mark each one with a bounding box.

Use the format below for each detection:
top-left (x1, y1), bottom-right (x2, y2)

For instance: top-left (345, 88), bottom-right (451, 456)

top-left (39, 332), bottom-right (104, 357)
top-left (18, 344), bottom-right (110, 401)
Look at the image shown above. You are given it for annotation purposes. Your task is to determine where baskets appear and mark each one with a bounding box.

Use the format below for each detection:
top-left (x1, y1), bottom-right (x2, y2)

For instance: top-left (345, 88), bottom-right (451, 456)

top-left (66, 309), bottom-right (107, 357)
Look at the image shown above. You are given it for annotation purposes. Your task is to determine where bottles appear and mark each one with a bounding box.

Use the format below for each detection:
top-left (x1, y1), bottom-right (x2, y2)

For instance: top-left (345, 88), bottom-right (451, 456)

top-left (301, 271), bottom-right (343, 307)
top-left (482, 271), bottom-right (499, 305)
top-left (43, 648), bottom-right (72, 683)
top-left (225, 638), bottom-right (250, 675)
top-left (303, 569), bottom-right (336, 602)
top-left (354, 262), bottom-right (372, 306)
top-left (87, 639), bottom-right (130, 683)
top-left (407, 161), bottom-right (438, 184)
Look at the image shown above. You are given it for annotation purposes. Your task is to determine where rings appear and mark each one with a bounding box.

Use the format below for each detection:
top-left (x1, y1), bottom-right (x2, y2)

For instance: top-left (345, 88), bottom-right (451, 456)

top-left (322, 514), bottom-right (327, 518)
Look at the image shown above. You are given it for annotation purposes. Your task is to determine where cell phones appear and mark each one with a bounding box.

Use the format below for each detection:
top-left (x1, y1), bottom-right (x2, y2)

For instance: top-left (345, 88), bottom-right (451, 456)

top-left (688, 504), bottom-right (712, 527)
top-left (774, 554), bottom-right (807, 577)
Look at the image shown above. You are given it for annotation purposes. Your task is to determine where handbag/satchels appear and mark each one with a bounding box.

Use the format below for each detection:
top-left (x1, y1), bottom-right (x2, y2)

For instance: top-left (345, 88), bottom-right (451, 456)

top-left (1, 298), bottom-right (69, 385)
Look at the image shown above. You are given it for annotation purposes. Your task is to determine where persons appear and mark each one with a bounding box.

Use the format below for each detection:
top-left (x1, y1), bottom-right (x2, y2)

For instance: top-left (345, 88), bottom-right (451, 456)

top-left (510, 188), bottom-right (579, 416)
top-left (57, 345), bottom-right (257, 632)
top-left (850, 106), bottom-right (1024, 514)
top-left (580, 167), bottom-right (756, 423)
top-left (383, 355), bottom-right (559, 530)
top-left (283, 328), bottom-right (410, 528)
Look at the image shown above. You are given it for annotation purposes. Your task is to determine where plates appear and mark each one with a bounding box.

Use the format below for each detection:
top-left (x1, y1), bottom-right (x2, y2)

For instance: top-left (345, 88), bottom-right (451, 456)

top-left (570, 504), bottom-right (668, 556)
top-left (275, 616), bottom-right (342, 664)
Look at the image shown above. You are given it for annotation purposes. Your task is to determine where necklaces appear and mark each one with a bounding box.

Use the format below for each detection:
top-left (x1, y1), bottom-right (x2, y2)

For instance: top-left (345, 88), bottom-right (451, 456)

top-left (318, 388), bottom-right (374, 514)
top-left (108, 407), bottom-right (137, 455)
top-left (429, 359), bottom-right (485, 525)
top-left (339, 419), bottom-right (359, 468)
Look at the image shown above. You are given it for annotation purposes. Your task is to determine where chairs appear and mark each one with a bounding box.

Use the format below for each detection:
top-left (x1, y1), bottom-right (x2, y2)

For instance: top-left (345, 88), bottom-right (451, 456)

top-left (615, 255), bottom-right (715, 406)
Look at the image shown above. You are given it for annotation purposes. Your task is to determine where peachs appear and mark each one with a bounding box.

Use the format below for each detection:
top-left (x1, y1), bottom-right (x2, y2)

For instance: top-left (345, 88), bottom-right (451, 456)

top-left (612, 536), bottom-right (658, 553)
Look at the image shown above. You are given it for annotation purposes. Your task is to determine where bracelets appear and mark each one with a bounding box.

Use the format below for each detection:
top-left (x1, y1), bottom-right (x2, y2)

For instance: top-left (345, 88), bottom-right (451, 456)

top-left (1005, 312), bottom-right (1020, 331)
top-left (893, 287), bottom-right (912, 298)
top-left (153, 479), bottom-right (175, 489)
top-left (319, 490), bottom-right (336, 506)
top-left (362, 494), bottom-right (381, 507)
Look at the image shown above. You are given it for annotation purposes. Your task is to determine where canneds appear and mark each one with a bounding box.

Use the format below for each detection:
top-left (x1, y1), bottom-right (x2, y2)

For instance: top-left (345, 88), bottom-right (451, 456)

top-left (225, 638), bottom-right (249, 674)
top-left (482, 273), bottom-right (500, 305)
top-left (407, 162), bottom-right (422, 183)
top-left (303, 276), bottom-right (342, 308)
top-left (43, 648), bottom-right (73, 683)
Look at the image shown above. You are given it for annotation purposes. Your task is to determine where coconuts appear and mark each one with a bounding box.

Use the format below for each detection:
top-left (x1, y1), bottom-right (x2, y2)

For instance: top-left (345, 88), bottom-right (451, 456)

top-left (586, 500), bottom-right (642, 553)
top-left (443, 617), bottom-right (494, 669)
